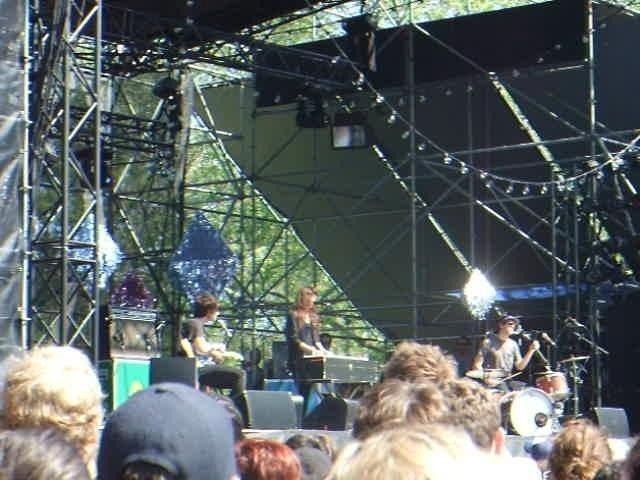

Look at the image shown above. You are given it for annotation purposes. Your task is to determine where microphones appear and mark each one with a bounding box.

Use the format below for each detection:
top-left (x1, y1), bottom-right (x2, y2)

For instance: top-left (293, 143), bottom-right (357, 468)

top-left (540, 330), bottom-right (559, 348)
top-left (215, 317), bottom-right (233, 339)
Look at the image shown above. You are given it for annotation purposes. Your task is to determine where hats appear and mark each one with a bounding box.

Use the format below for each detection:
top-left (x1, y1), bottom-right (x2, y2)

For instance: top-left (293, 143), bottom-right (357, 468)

top-left (98, 383), bottom-right (235, 479)
top-left (496, 316), bottom-right (520, 325)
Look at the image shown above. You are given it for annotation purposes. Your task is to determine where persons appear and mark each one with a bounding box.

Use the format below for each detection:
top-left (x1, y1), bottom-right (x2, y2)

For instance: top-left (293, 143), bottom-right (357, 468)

top-left (178, 293), bottom-right (253, 430)
top-left (285, 288), bottom-right (328, 396)
top-left (0, 343), bottom-right (640, 479)
top-left (474, 316), bottom-right (541, 377)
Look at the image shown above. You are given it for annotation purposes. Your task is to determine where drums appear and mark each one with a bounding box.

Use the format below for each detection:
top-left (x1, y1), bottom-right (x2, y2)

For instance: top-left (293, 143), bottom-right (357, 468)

top-left (499, 386), bottom-right (556, 434)
top-left (536, 372), bottom-right (570, 401)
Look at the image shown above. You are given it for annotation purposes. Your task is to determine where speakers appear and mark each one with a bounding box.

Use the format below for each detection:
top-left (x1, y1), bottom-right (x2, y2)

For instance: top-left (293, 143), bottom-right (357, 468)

top-left (301, 395), bottom-right (360, 430)
top-left (231, 389), bottom-right (297, 429)
top-left (584, 406), bottom-right (631, 437)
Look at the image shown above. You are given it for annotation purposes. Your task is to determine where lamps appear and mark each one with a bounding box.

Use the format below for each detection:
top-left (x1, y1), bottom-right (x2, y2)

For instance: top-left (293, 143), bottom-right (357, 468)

top-left (251, 11), bottom-right (381, 128)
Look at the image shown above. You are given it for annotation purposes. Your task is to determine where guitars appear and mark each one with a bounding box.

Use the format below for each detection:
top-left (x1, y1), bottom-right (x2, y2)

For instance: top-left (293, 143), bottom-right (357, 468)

top-left (181, 341), bottom-right (244, 366)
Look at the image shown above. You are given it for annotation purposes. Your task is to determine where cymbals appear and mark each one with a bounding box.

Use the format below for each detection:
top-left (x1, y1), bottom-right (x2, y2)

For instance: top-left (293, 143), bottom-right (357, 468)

top-left (560, 355), bottom-right (590, 363)
top-left (465, 368), bottom-right (507, 379)
top-left (535, 371), bottom-right (555, 375)
